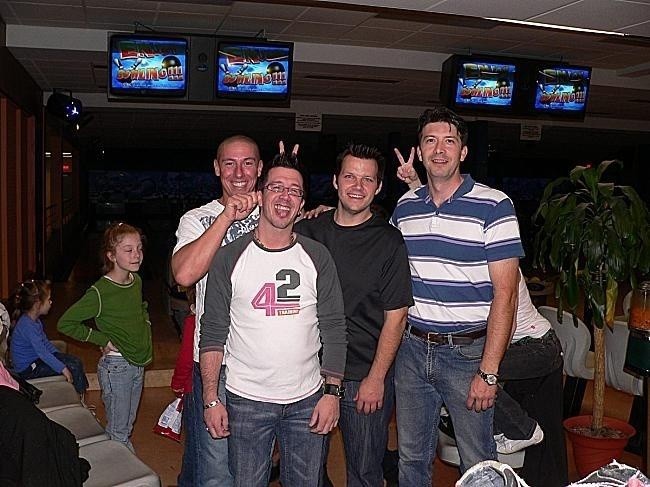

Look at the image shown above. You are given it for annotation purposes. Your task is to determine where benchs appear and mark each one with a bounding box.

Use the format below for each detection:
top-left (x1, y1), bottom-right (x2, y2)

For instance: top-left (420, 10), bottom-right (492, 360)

top-left (26, 341), bottom-right (160, 487)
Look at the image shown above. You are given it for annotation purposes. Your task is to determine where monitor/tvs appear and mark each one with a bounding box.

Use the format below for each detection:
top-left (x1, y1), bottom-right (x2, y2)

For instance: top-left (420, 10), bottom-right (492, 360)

top-left (212, 34), bottom-right (294, 109)
top-left (441, 53), bottom-right (517, 117)
top-left (107, 37), bottom-right (190, 102)
top-left (533, 65), bottom-right (591, 121)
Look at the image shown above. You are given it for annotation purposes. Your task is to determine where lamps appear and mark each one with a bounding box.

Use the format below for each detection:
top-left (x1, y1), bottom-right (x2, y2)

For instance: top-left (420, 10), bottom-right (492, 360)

top-left (48, 87), bottom-right (82, 125)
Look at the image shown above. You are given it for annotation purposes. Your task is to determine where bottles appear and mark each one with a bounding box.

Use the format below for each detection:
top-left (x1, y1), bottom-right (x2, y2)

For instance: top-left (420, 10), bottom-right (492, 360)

top-left (628, 280), bottom-right (650, 330)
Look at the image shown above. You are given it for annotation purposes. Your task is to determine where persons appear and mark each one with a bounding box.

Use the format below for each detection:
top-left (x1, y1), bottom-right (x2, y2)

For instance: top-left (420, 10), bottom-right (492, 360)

top-left (54, 219), bottom-right (154, 445)
top-left (277, 138), bottom-right (415, 485)
top-left (172, 132), bottom-right (307, 486)
top-left (302, 107), bottom-right (526, 486)
top-left (0, 299), bottom-right (20, 391)
top-left (391, 141), bottom-right (568, 486)
top-left (12, 276), bottom-right (95, 408)
top-left (196, 150), bottom-right (348, 485)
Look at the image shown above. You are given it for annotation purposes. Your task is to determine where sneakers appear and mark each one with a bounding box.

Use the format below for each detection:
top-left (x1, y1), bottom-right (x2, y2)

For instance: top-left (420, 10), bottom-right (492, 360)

top-left (494, 421), bottom-right (544, 455)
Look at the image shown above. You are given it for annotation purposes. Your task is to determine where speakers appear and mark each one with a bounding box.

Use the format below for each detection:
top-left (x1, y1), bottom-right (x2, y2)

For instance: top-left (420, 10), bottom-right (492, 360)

top-left (47, 91), bottom-right (83, 124)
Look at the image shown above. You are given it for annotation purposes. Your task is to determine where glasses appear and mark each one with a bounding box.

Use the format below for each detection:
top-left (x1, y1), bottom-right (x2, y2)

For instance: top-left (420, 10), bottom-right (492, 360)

top-left (262, 183), bottom-right (304, 197)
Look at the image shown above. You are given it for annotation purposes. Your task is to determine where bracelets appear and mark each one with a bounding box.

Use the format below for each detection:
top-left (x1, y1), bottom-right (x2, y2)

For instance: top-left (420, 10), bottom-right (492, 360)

top-left (202, 396), bottom-right (222, 407)
top-left (322, 382), bottom-right (347, 399)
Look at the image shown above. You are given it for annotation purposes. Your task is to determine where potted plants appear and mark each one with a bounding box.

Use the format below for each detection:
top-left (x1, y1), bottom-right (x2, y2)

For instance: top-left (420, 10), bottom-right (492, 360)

top-left (534, 159), bottom-right (650, 477)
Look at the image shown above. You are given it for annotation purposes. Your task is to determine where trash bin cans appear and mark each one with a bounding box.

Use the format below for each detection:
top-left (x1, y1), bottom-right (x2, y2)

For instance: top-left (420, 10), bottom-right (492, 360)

top-left (526, 281), bottom-right (554, 307)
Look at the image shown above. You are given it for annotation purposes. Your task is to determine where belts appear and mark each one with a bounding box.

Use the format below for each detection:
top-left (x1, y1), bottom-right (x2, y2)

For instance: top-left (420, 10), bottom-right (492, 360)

top-left (405, 322), bottom-right (487, 345)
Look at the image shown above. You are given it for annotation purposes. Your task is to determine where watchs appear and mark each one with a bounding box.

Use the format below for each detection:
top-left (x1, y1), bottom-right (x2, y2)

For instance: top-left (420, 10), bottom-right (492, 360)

top-left (476, 366), bottom-right (501, 386)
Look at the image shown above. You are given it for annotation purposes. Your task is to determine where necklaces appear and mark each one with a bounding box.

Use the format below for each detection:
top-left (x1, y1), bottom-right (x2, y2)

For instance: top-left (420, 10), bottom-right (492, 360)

top-left (251, 229), bottom-right (296, 250)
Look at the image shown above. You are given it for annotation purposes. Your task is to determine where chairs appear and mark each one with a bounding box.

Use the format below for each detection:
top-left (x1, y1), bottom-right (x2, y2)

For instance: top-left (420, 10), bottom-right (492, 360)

top-left (435, 305), bottom-right (643, 469)
